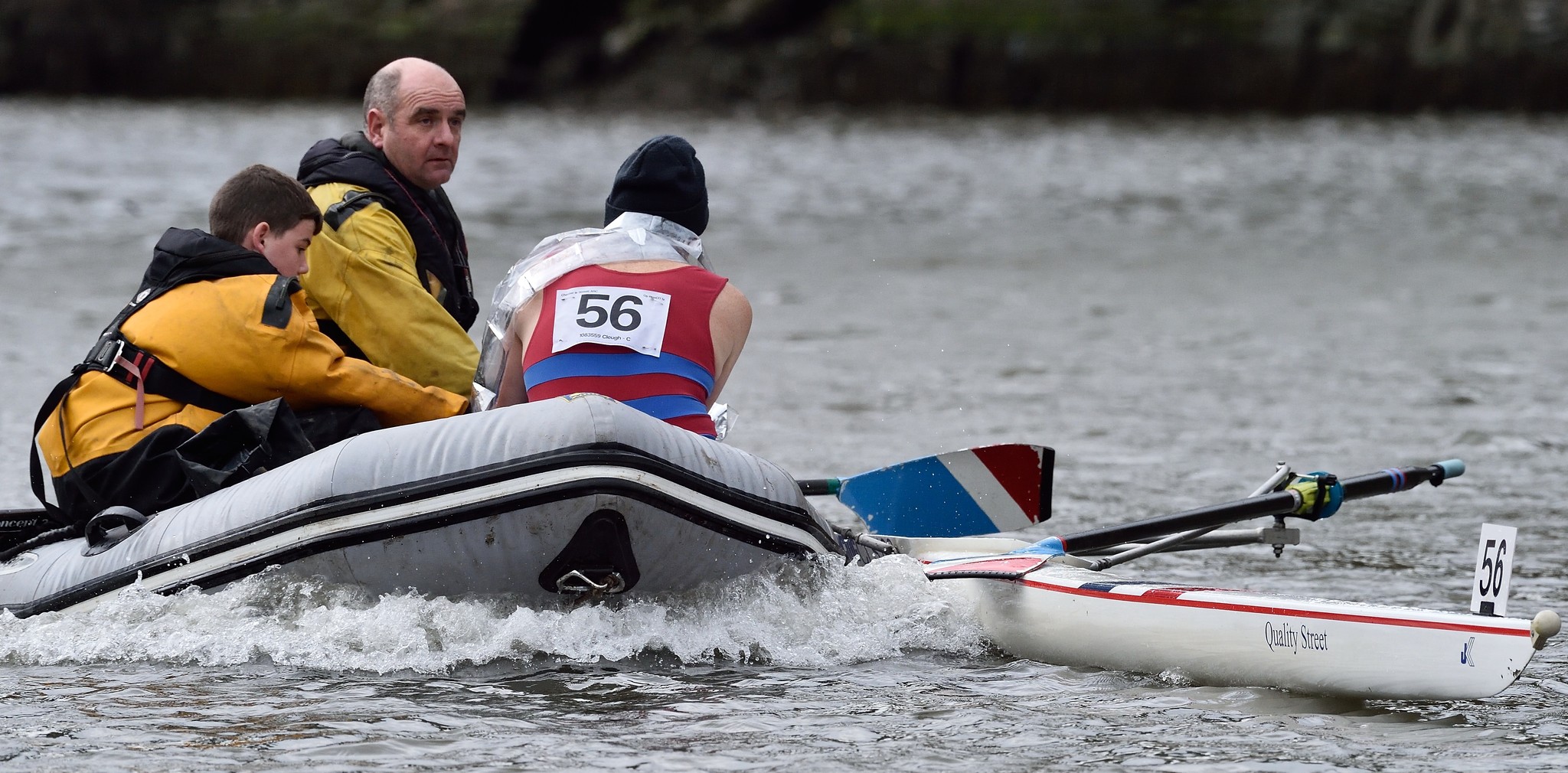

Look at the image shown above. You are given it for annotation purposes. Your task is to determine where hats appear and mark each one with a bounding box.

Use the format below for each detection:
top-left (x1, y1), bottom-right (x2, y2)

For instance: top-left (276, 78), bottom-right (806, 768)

top-left (604, 135), bottom-right (710, 240)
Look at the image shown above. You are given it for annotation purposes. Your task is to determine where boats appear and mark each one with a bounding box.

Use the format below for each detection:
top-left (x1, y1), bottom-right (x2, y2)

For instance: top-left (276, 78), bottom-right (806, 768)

top-left (923, 551), bottom-right (1560, 702)
top-left (0, 392), bottom-right (856, 668)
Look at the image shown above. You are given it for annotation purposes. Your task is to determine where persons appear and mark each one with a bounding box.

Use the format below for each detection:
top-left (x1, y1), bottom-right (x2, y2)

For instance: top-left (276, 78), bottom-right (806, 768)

top-left (292, 53), bottom-right (485, 420)
top-left (30, 163), bottom-right (469, 514)
top-left (490, 133), bottom-right (752, 440)
